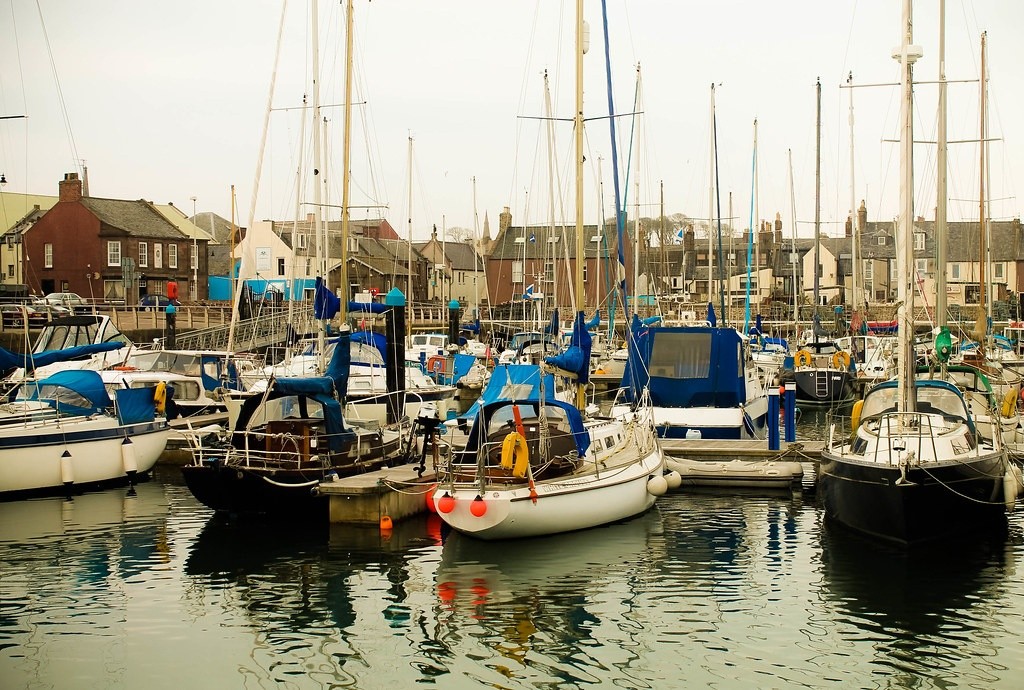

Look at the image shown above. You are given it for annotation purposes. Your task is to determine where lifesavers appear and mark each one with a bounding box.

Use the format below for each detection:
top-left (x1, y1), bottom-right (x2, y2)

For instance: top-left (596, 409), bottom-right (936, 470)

top-left (794, 350), bottom-right (812, 369)
top-left (426, 354), bottom-right (447, 374)
top-left (851, 400), bottom-right (865, 433)
top-left (500, 431), bottom-right (531, 479)
top-left (833, 350), bottom-right (851, 368)
top-left (154, 381), bottom-right (167, 413)
top-left (1001, 388), bottom-right (1018, 418)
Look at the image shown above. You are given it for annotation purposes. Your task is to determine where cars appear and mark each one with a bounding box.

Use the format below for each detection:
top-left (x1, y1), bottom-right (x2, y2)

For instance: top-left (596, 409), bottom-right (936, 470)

top-left (138, 294), bottom-right (182, 311)
top-left (755, 300), bottom-right (796, 322)
top-left (34, 292), bottom-right (87, 315)
top-left (1, 304), bottom-right (37, 327)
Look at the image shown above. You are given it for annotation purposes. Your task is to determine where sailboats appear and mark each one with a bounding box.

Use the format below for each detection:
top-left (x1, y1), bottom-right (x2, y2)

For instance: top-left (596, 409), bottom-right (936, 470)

top-left (0, 0), bottom-right (1024, 539)
